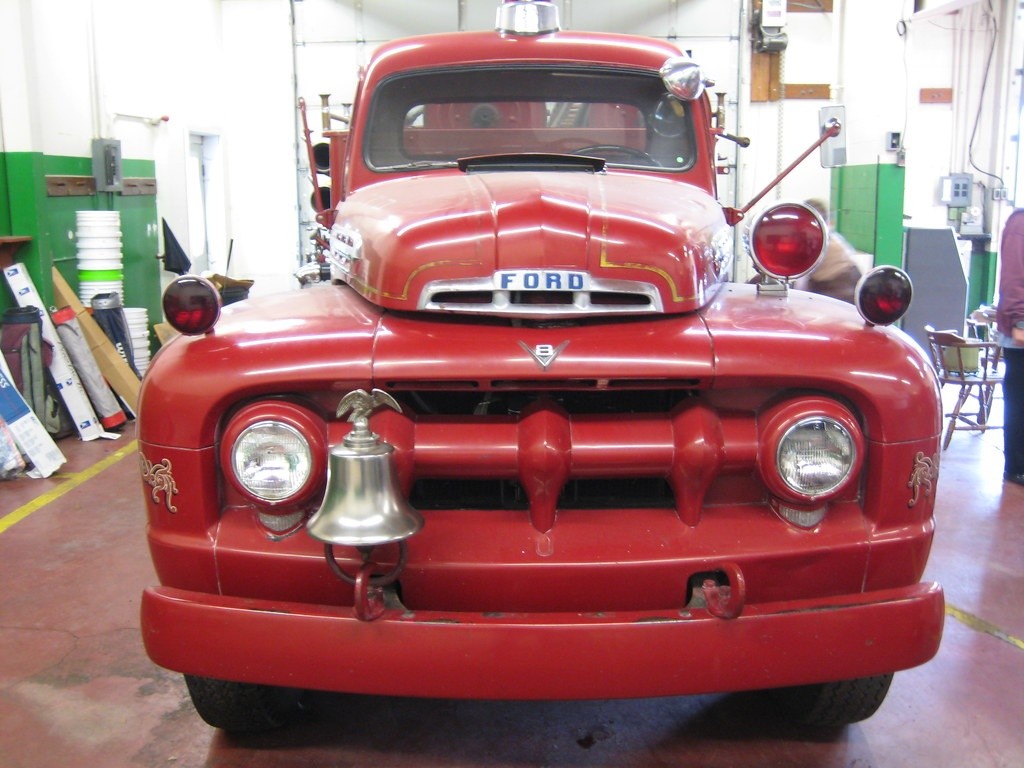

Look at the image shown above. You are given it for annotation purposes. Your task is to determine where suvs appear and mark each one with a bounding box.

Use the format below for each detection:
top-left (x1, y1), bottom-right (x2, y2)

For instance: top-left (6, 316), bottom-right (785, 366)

top-left (137, 0), bottom-right (944, 737)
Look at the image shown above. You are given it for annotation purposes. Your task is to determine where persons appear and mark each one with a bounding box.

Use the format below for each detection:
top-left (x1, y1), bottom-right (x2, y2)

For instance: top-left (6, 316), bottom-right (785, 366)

top-left (995, 207), bottom-right (1024, 486)
top-left (800, 198), bottom-right (863, 305)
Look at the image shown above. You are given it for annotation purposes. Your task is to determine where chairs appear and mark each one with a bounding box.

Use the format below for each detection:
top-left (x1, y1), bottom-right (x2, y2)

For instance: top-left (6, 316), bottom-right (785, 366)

top-left (924, 325), bottom-right (1003, 450)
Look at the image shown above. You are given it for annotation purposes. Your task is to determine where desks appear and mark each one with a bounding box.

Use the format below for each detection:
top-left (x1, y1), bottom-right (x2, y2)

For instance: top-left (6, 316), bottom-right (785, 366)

top-left (965, 305), bottom-right (999, 430)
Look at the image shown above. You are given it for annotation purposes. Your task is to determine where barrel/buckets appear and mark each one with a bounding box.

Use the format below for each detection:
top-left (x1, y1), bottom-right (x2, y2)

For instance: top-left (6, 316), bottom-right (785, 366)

top-left (218, 283), bottom-right (250, 306)
top-left (74, 210), bottom-right (151, 380)
